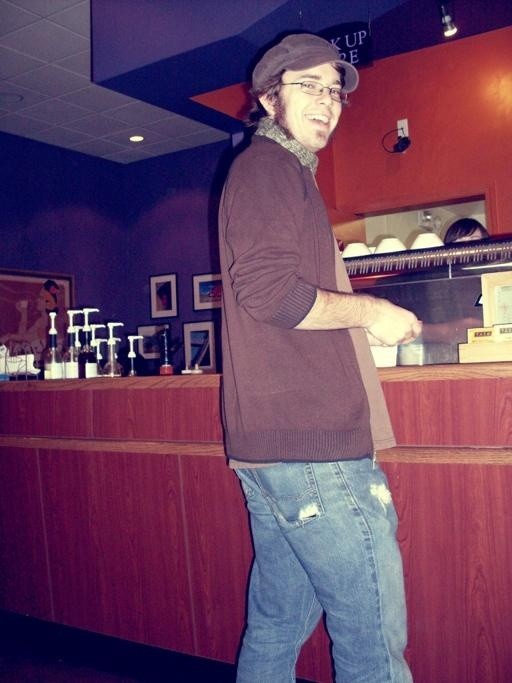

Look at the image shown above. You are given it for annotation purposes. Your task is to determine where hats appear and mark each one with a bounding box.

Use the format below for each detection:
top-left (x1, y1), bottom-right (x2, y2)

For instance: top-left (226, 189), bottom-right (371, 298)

top-left (252, 33), bottom-right (359, 93)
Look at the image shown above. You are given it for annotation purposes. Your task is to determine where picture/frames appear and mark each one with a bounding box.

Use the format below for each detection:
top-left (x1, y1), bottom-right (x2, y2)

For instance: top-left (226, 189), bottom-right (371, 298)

top-left (0, 267), bottom-right (76, 362)
top-left (183, 320), bottom-right (216, 374)
top-left (150, 273), bottom-right (178, 319)
top-left (193, 272), bottom-right (222, 310)
top-left (137, 323), bottom-right (171, 360)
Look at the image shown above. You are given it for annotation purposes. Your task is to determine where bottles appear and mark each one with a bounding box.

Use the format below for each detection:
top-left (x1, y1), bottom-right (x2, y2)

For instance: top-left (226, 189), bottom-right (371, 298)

top-left (399, 320), bottom-right (424, 367)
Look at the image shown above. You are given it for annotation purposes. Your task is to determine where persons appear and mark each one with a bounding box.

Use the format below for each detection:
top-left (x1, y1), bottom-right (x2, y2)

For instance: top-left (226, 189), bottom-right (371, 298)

top-left (400, 218), bottom-right (491, 345)
top-left (218, 33), bottom-right (423, 682)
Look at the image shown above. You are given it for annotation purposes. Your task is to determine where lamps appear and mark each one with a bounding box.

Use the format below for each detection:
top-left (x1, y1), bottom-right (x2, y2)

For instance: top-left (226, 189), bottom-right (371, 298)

top-left (440, 5), bottom-right (457, 37)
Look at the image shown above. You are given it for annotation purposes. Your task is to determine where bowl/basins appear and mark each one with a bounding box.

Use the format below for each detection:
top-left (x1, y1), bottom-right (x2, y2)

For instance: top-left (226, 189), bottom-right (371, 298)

top-left (340, 242), bottom-right (372, 259)
top-left (409, 232), bottom-right (446, 250)
top-left (374, 237), bottom-right (408, 255)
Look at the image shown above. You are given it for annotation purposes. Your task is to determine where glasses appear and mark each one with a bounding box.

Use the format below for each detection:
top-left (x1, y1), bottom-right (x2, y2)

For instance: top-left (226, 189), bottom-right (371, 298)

top-left (281, 80), bottom-right (348, 105)
